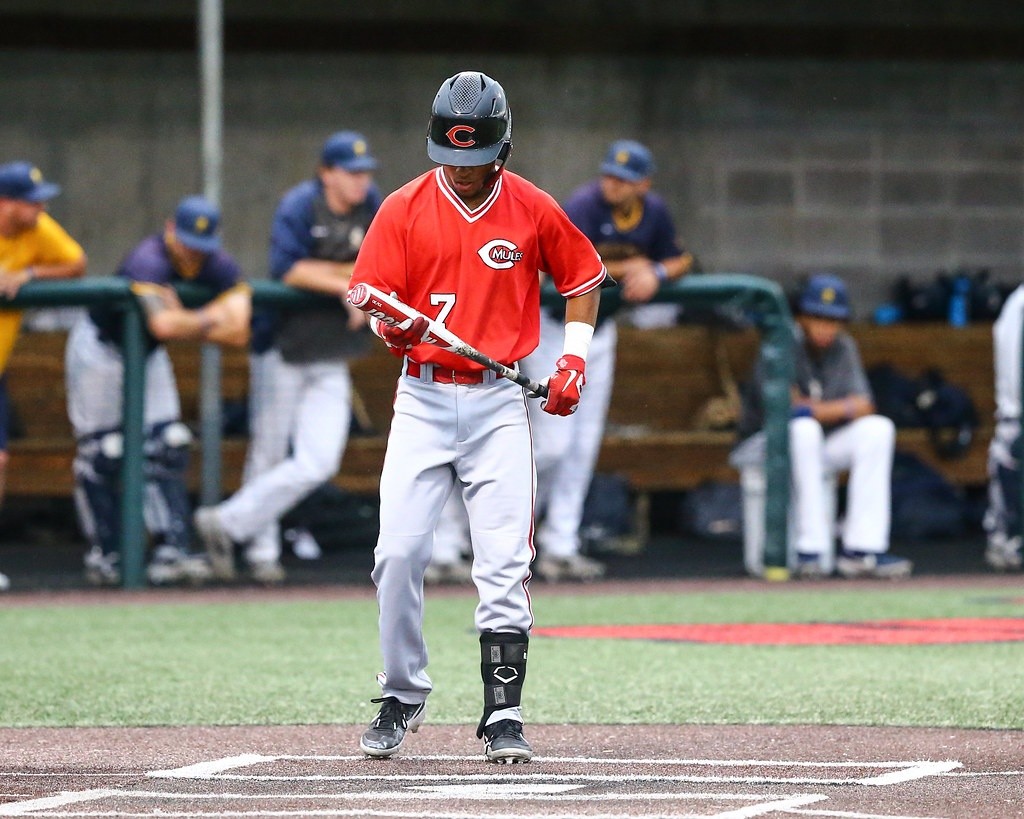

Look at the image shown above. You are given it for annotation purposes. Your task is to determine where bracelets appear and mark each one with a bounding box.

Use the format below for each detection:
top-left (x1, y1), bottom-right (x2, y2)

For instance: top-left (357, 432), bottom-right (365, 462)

top-left (648, 260), bottom-right (670, 286)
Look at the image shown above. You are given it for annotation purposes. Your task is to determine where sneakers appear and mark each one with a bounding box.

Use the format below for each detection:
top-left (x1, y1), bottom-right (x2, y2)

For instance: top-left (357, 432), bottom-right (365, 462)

top-left (837, 554), bottom-right (912, 578)
top-left (424, 561), bottom-right (475, 584)
top-left (359, 695), bottom-right (427, 756)
top-left (795, 562), bottom-right (823, 581)
top-left (247, 562), bottom-right (287, 583)
top-left (482, 719), bottom-right (532, 764)
top-left (535, 552), bottom-right (607, 581)
top-left (191, 506), bottom-right (239, 583)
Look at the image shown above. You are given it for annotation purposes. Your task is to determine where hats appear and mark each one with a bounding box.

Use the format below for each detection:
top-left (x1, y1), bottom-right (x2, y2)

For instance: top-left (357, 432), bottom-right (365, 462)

top-left (800, 275), bottom-right (849, 320)
top-left (602, 140), bottom-right (656, 184)
top-left (321, 132), bottom-right (377, 173)
top-left (0, 161), bottom-right (62, 203)
top-left (175, 195), bottom-right (221, 254)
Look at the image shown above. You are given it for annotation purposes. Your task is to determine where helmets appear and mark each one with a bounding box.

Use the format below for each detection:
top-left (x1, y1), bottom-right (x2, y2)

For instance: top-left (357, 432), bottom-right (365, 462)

top-left (426, 72), bottom-right (514, 167)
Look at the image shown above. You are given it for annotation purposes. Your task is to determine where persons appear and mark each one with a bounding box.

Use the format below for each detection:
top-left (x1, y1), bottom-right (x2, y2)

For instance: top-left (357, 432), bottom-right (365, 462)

top-left (418, 138), bottom-right (694, 587)
top-left (979, 281), bottom-right (1024, 575)
top-left (0, 160), bottom-right (89, 594)
top-left (345, 70), bottom-right (618, 765)
top-left (62, 196), bottom-right (255, 590)
top-left (191, 131), bottom-right (385, 590)
top-left (728, 270), bottom-right (915, 581)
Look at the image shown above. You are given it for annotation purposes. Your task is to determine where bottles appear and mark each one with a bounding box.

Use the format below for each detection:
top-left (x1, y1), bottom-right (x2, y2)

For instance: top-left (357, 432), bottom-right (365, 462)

top-left (951, 276), bottom-right (972, 328)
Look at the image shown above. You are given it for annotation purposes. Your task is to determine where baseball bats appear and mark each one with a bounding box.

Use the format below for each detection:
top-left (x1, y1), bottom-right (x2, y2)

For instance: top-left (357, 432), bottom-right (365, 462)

top-left (347, 281), bottom-right (580, 413)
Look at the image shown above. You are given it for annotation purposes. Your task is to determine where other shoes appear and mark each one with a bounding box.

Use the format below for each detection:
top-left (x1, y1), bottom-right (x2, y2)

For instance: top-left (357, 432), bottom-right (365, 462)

top-left (0, 572), bottom-right (10, 592)
top-left (149, 558), bottom-right (212, 584)
top-left (86, 552), bottom-right (119, 585)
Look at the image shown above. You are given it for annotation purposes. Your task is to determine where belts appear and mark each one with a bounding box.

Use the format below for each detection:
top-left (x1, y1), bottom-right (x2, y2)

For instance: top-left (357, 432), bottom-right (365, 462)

top-left (407, 361), bottom-right (515, 386)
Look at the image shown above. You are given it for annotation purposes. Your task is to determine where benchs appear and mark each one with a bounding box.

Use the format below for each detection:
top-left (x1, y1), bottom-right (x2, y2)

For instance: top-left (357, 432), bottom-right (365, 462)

top-left (0, 321), bottom-right (994, 536)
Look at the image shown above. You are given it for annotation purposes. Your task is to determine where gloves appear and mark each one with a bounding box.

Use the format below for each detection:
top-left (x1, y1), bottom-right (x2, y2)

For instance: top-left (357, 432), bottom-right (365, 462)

top-left (527, 354), bottom-right (587, 416)
top-left (376, 291), bottom-right (430, 359)
